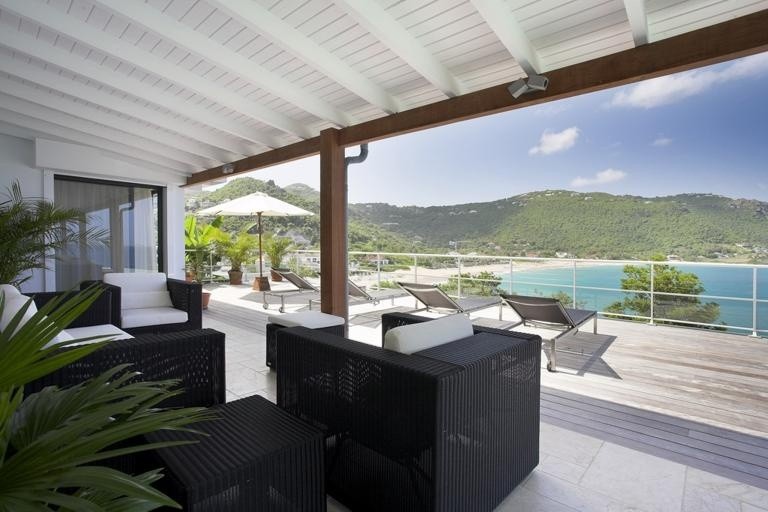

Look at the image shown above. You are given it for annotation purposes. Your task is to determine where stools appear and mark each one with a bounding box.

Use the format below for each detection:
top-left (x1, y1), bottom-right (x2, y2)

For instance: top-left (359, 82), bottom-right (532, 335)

top-left (135, 394), bottom-right (326, 512)
top-left (267, 308), bottom-right (345, 369)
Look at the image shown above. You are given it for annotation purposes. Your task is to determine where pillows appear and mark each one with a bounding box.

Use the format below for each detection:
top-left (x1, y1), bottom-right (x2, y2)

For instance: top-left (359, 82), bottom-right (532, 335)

top-left (1, 284), bottom-right (77, 349)
top-left (383, 314), bottom-right (474, 355)
top-left (103, 272), bottom-right (173, 310)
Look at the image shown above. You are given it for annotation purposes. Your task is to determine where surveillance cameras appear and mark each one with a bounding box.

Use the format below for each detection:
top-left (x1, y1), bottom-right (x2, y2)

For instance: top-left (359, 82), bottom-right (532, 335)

top-left (506, 77), bottom-right (526, 99)
top-left (527, 75), bottom-right (549, 91)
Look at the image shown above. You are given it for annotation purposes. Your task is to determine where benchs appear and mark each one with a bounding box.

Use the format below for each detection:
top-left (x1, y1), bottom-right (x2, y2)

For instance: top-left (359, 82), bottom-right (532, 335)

top-left (0, 284), bottom-right (225, 412)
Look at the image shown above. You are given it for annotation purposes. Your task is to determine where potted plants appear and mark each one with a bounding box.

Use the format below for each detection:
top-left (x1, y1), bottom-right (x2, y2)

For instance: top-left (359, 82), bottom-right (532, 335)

top-left (185, 213), bottom-right (223, 309)
top-left (220, 223), bottom-right (257, 284)
top-left (261, 237), bottom-right (293, 281)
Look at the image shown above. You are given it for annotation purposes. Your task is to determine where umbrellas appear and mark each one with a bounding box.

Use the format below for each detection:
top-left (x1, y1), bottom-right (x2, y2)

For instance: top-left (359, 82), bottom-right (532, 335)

top-left (196, 191), bottom-right (315, 277)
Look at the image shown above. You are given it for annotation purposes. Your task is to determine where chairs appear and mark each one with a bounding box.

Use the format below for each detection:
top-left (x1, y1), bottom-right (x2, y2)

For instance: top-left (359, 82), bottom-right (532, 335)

top-left (497, 290), bottom-right (598, 370)
top-left (276, 313), bottom-right (540, 511)
top-left (263, 267), bottom-right (367, 314)
top-left (80, 273), bottom-right (202, 335)
top-left (310, 270), bottom-right (411, 310)
top-left (397, 281), bottom-right (503, 321)
